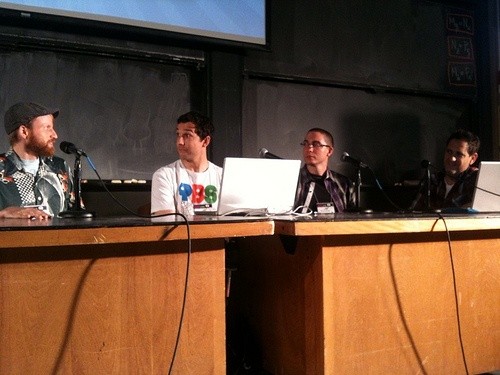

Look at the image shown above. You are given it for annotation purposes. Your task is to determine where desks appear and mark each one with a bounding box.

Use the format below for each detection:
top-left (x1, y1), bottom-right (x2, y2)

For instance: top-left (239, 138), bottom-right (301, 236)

top-left (0, 217), bottom-right (277, 375)
top-left (260, 212), bottom-right (500, 375)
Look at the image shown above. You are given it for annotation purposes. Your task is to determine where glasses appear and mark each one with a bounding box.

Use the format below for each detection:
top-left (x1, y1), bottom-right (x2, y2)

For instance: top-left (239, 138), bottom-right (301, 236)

top-left (300, 141), bottom-right (331, 148)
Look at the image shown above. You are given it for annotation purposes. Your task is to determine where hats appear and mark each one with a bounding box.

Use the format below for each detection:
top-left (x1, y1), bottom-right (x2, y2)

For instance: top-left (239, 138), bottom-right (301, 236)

top-left (4, 101), bottom-right (60, 135)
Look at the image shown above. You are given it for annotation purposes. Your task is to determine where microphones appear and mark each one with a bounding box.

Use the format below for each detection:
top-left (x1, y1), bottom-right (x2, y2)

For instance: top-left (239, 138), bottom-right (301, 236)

top-left (259, 147), bottom-right (283, 159)
top-left (340, 152), bottom-right (372, 171)
top-left (60, 141), bottom-right (86, 156)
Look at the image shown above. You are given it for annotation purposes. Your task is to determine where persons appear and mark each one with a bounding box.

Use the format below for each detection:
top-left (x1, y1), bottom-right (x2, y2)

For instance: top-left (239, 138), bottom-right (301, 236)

top-left (151, 110), bottom-right (223, 217)
top-left (409, 130), bottom-right (480, 213)
top-left (293, 127), bottom-right (358, 213)
top-left (0, 100), bottom-right (85, 220)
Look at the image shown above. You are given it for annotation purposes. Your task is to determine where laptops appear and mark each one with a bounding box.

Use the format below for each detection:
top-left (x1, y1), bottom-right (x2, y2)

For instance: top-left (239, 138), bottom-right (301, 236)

top-left (471, 161), bottom-right (500, 213)
top-left (194, 157), bottom-right (301, 216)
